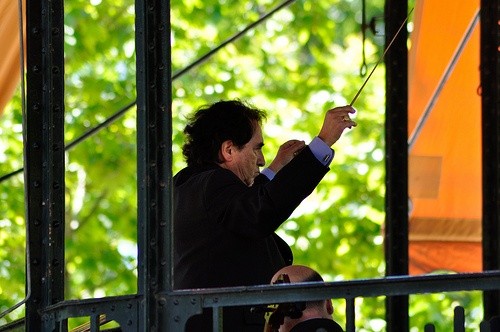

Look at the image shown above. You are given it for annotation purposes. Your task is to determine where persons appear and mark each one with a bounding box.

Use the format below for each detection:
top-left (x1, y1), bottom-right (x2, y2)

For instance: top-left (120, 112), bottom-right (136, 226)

top-left (270, 265), bottom-right (344, 332)
top-left (173, 99), bottom-right (357, 332)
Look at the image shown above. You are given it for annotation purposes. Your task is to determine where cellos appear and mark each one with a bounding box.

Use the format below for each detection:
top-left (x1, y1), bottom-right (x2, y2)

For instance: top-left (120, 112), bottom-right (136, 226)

top-left (250, 274), bottom-right (305, 332)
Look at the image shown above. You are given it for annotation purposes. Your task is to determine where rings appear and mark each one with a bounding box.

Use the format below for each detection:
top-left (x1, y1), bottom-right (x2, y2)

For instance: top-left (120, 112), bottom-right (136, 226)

top-left (342, 116), bottom-right (346, 122)
